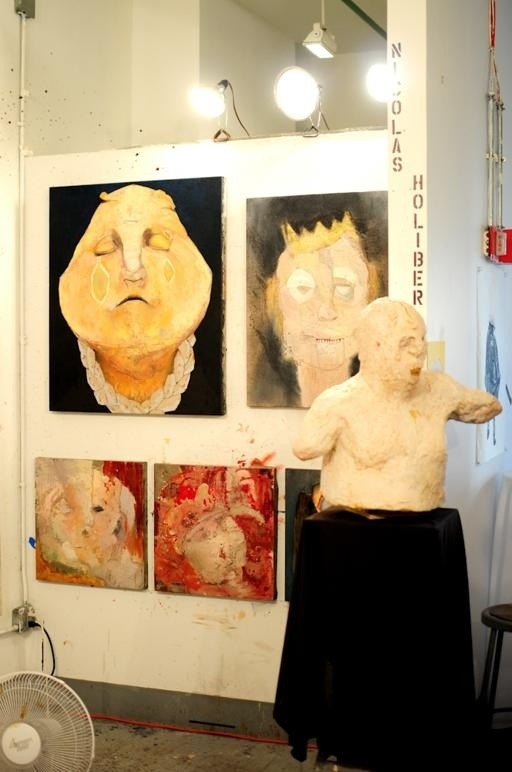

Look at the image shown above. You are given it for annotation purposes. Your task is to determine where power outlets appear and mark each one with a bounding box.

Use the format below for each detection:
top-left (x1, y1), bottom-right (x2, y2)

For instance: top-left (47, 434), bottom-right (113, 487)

top-left (13, 604), bottom-right (36, 633)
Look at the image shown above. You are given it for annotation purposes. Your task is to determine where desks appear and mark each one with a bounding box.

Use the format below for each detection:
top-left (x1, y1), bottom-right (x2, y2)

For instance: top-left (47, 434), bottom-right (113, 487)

top-left (275, 509), bottom-right (482, 771)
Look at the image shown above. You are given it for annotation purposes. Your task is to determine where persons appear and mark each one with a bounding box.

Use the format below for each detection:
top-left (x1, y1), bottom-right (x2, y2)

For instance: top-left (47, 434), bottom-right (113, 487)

top-left (293, 297), bottom-right (503, 512)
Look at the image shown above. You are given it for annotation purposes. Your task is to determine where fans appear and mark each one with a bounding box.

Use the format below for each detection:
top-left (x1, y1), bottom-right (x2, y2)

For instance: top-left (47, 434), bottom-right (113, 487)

top-left (1, 676), bottom-right (95, 770)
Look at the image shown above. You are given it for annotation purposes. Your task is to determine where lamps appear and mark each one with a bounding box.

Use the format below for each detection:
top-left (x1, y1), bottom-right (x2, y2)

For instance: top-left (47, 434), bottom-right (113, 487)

top-left (273, 1), bottom-right (338, 131)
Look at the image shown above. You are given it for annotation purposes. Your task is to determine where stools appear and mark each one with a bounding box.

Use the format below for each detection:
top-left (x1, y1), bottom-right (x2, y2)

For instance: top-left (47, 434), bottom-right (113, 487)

top-left (483, 603), bottom-right (512, 741)
